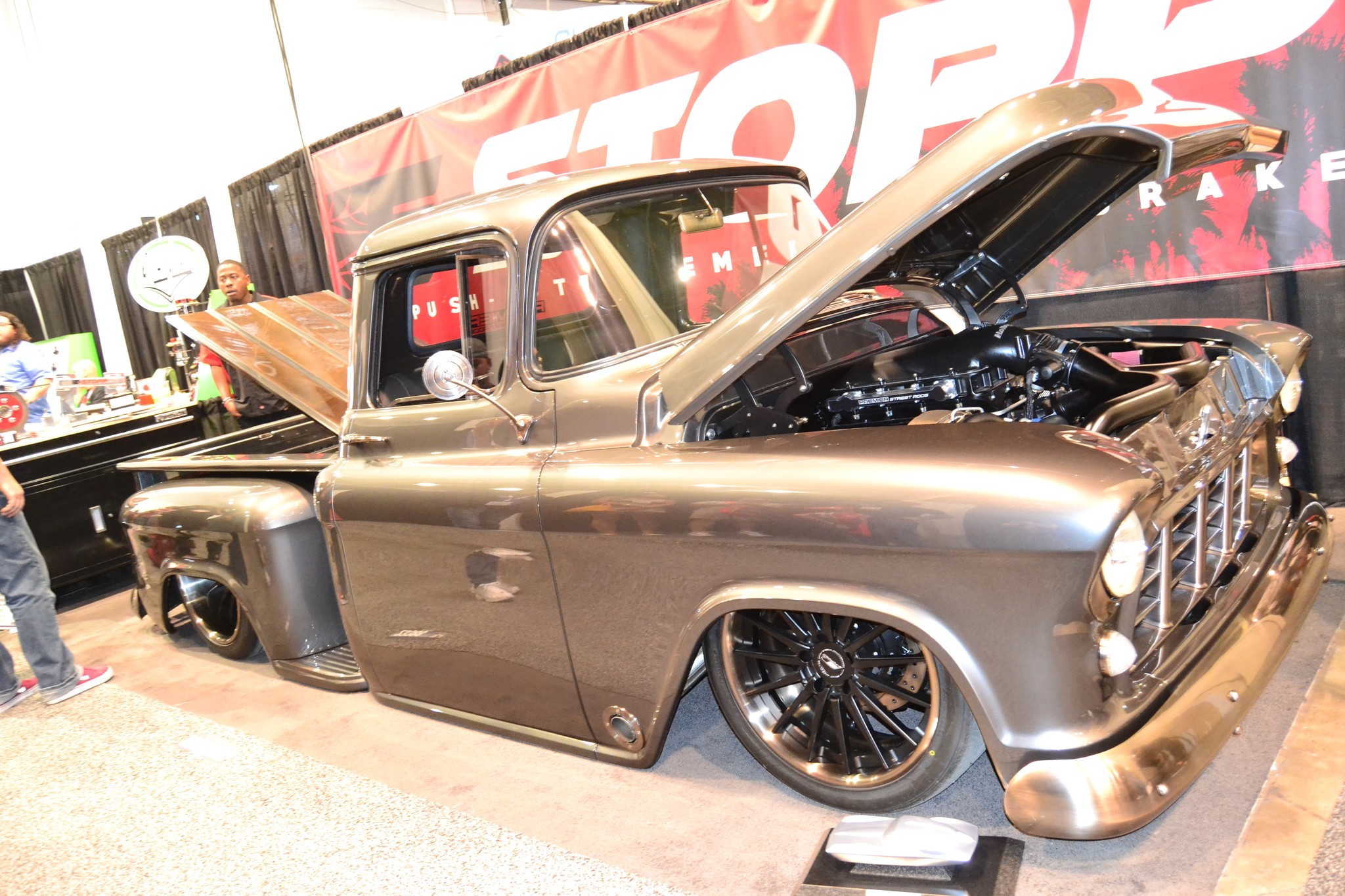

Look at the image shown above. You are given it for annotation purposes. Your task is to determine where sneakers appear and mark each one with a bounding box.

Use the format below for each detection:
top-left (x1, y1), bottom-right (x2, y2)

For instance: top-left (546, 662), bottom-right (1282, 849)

top-left (0, 676), bottom-right (41, 714)
top-left (47, 665), bottom-right (114, 705)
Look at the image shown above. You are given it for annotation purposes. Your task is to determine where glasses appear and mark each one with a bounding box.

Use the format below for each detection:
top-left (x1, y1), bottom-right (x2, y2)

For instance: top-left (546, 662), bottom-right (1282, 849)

top-left (0, 323), bottom-right (14, 326)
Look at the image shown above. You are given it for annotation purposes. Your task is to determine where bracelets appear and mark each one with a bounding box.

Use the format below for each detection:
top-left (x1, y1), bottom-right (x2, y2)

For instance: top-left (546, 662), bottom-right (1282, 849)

top-left (220, 396), bottom-right (233, 409)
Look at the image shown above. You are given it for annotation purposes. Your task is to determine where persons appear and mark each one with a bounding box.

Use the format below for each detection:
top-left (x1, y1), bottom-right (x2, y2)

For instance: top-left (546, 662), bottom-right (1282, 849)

top-left (195, 260), bottom-right (294, 429)
top-left (0, 310), bottom-right (57, 424)
top-left (1, 433), bottom-right (115, 713)
top-left (188, 361), bottom-right (240, 439)
top-left (452, 338), bottom-right (495, 389)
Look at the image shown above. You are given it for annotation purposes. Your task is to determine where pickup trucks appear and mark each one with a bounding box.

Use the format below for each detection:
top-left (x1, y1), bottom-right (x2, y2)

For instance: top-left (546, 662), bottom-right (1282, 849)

top-left (112, 71), bottom-right (1338, 844)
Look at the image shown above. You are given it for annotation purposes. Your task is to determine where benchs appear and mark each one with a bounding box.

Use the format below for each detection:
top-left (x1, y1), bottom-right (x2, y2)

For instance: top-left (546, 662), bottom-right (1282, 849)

top-left (375, 315), bottom-right (626, 406)
